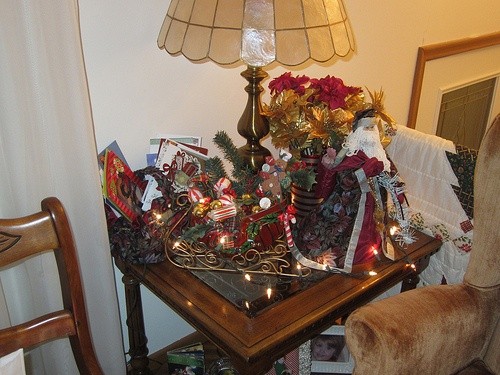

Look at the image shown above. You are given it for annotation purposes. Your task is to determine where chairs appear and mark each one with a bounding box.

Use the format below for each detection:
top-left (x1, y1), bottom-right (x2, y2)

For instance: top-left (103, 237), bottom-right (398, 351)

top-left (0, 196), bottom-right (104, 375)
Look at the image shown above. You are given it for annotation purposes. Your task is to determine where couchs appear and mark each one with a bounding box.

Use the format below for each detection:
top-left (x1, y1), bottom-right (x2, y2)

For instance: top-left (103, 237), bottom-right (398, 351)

top-left (343, 112), bottom-right (500, 375)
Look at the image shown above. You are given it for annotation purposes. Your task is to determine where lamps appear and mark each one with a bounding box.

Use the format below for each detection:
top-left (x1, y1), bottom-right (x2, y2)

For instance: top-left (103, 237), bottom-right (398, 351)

top-left (156, 0), bottom-right (356, 176)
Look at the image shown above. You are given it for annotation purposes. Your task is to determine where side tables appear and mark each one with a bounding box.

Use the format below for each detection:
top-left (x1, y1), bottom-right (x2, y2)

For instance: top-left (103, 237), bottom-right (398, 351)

top-left (103, 212), bottom-right (443, 375)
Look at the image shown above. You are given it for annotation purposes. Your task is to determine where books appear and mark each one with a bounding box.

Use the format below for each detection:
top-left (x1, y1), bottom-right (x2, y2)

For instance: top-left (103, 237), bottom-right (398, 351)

top-left (166, 342), bottom-right (205, 375)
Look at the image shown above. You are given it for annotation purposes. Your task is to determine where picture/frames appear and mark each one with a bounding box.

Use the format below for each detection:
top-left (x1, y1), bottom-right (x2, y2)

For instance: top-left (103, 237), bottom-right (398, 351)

top-left (406, 32), bottom-right (500, 151)
top-left (310, 326), bottom-right (354, 374)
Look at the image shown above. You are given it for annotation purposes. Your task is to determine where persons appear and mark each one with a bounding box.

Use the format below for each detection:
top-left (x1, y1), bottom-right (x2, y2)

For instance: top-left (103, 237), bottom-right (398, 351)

top-left (308, 335), bottom-right (346, 362)
top-left (274, 356), bottom-right (293, 375)
top-left (317, 110), bottom-right (406, 261)
